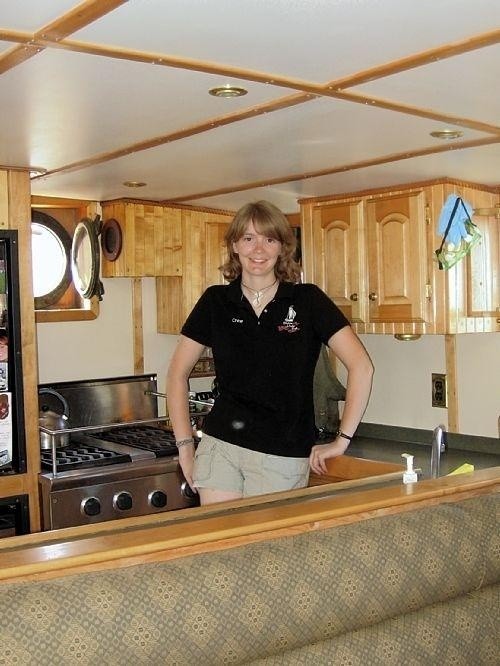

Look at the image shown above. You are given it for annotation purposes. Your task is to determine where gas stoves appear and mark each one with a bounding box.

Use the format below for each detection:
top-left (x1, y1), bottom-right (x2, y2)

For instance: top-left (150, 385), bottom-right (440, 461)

top-left (36, 423), bottom-right (201, 532)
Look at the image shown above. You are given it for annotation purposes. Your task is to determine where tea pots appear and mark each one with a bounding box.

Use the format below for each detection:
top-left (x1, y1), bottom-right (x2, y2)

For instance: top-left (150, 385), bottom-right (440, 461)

top-left (37, 388), bottom-right (71, 451)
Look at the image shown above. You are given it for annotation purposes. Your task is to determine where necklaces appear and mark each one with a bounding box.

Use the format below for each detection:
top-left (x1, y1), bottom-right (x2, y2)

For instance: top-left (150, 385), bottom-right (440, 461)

top-left (240, 280), bottom-right (279, 309)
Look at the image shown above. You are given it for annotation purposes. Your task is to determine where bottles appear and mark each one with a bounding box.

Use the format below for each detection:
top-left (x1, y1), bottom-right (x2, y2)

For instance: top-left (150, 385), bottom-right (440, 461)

top-left (190, 395), bottom-right (198, 430)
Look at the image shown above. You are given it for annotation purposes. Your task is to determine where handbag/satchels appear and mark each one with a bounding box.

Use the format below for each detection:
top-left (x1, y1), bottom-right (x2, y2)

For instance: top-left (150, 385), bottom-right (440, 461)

top-left (435, 218), bottom-right (481, 271)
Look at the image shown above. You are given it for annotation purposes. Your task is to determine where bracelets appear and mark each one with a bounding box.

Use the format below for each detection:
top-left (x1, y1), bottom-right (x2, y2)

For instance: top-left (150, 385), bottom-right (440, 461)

top-left (175, 438), bottom-right (194, 447)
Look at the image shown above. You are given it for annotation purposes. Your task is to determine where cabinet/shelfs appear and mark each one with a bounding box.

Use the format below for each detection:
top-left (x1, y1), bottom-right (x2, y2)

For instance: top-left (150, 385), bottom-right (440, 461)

top-left (297, 176), bottom-right (498, 335)
top-left (100, 197), bottom-right (236, 334)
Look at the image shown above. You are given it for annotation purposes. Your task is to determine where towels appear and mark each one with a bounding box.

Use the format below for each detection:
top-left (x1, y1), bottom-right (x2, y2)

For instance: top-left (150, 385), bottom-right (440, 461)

top-left (437, 194), bottom-right (473, 246)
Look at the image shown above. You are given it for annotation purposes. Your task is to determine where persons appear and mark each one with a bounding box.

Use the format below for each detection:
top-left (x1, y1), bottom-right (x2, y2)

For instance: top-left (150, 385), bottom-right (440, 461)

top-left (166, 199), bottom-right (375, 506)
top-left (0, 334), bottom-right (8, 361)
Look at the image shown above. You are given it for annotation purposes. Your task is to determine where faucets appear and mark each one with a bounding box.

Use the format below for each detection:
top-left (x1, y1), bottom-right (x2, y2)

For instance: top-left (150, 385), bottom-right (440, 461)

top-left (430, 425), bottom-right (446, 479)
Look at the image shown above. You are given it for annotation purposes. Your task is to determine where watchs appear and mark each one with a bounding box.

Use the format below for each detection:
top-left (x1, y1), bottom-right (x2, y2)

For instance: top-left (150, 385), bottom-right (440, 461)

top-left (336, 430), bottom-right (352, 440)
